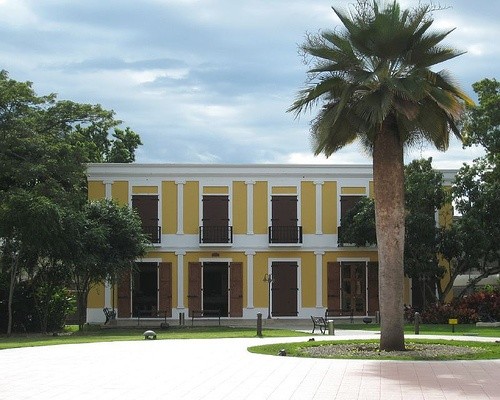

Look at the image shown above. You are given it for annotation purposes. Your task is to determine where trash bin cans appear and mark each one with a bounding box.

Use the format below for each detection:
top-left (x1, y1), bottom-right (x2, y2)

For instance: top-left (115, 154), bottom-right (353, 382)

top-left (327, 320), bottom-right (335, 335)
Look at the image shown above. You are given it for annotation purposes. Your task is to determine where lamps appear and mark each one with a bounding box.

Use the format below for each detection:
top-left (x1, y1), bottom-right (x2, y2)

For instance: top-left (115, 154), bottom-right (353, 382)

top-left (263, 273), bottom-right (268, 283)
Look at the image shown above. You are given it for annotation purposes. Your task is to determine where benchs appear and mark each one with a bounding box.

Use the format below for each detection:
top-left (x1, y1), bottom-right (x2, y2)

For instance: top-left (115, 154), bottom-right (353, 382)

top-left (326, 309), bottom-right (354, 326)
top-left (192, 310), bottom-right (221, 326)
top-left (138, 309), bottom-right (166, 328)
top-left (311, 316), bottom-right (328, 335)
